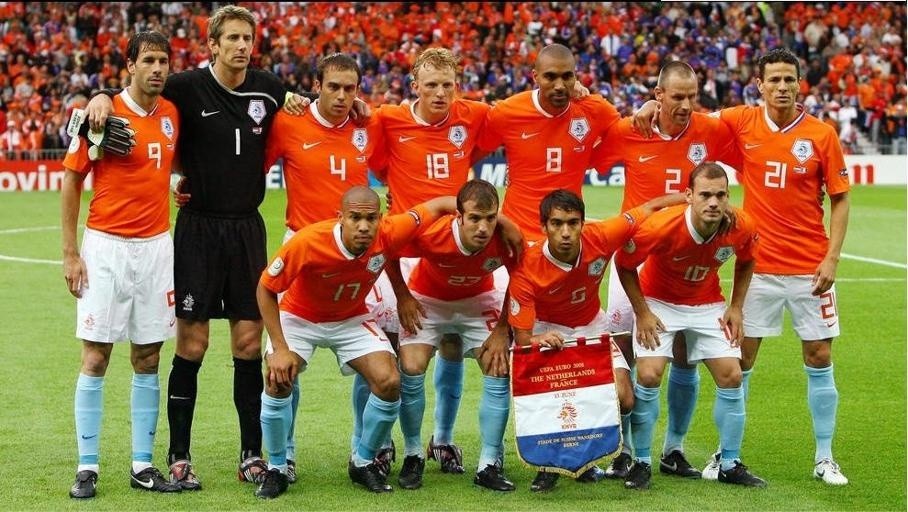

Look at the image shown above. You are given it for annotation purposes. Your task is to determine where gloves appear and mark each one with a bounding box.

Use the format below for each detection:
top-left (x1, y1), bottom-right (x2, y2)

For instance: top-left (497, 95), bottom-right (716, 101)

top-left (64, 107), bottom-right (137, 159)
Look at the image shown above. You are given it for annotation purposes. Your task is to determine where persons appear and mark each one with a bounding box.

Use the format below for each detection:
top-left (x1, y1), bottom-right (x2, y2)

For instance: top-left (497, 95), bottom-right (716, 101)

top-left (379, 175), bottom-right (526, 495)
top-left (79, 3), bottom-right (374, 489)
top-left (282, 44), bottom-right (592, 476)
top-left (251, 184), bottom-right (530, 498)
top-left (171, 53), bottom-right (398, 485)
top-left (501, 59), bottom-right (826, 477)
top-left (611, 159), bottom-right (772, 492)
top-left (467, 42), bottom-right (625, 253)
top-left (1, 1), bottom-right (907, 160)
top-left (58, 28), bottom-right (185, 500)
top-left (504, 186), bottom-right (738, 492)
top-left (628, 45), bottom-right (854, 489)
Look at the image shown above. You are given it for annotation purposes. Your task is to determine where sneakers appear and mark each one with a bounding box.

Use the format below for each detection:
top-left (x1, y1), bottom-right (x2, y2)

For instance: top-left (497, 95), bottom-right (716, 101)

top-left (68, 469), bottom-right (97, 498)
top-left (576, 465), bottom-right (604, 483)
top-left (373, 439), bottom-right (396, 479)
top-left (168, 460), bottom-right (201, 491)
top-left (530, 470), bottom-right (560, 491)
top-left (812, 459), bottom-right (850, 487)
top-left (254, 468), bottom-right (289, 498)
top-left (658, 450), bottom-right (701, 479)
top-left (427, 435), bottom-right (464, 474)
top-left (702, 451), bottom-right (722, 480)
top-left (238, 457), bottom-right (268, 483)
top-left (605, 453), bottom-right (633, 477)
top-left (348, 461), bottom-right (392, 494)
top-left (474, 464), bottom-right (515, 491)
top-left (287, 460), bottom-right (296, 483)
top-left (130, 467), bottom-right (183, 493)
top-left (624, 461), bottom-right (652, 488)
top-left (398, 455), bottom-right (425, 490)
top-left (719, 460), bottom-right (766, 487)
